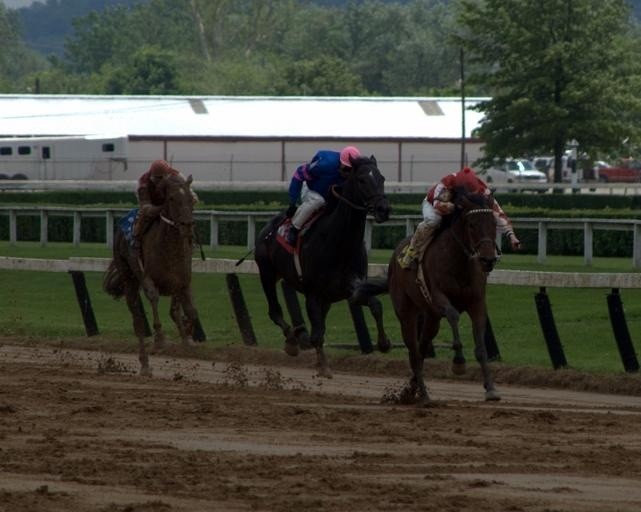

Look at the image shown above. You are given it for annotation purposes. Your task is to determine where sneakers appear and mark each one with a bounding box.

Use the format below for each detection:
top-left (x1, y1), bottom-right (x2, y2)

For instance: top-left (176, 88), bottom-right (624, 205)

top-left (130, 234), bottom-right (140, 249)
top-left (287, 224), bottom-right (299, 243)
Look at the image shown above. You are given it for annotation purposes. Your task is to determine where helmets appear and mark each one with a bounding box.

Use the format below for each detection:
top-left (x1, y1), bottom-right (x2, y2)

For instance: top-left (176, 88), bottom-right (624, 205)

top-left (456, 167), bottom-right (477, 187)
top-left (151, 160), bottom-right (171, 177)
top-left (340, 146), bottom-right (361, 167)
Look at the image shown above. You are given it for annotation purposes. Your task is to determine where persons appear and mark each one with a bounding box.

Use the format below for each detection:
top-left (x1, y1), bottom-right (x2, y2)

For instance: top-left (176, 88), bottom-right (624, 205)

top-left (398, 167), bottom-right (522, 270)
top-left (285, 146), bottom-right (362, 244)
top-left (127, 159), bottom-right (198, 258)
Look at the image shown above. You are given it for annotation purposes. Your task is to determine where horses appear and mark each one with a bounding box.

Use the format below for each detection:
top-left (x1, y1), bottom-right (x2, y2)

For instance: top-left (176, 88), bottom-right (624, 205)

top-left (102, 172), bottom-right (207, 376)
top-left (247, 151), bottom-right (394, 381)
top-left (385, 186), bottom-right (504, 408)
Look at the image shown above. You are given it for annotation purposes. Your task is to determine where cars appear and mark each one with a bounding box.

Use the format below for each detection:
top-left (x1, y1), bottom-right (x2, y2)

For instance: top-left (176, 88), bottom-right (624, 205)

top-left (477, 150), bottom-right (641, 193)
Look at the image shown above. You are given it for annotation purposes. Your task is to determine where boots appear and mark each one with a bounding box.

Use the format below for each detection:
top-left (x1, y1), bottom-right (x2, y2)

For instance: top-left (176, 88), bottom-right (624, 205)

top-left (404, 224), bottom-right (436, 264)
